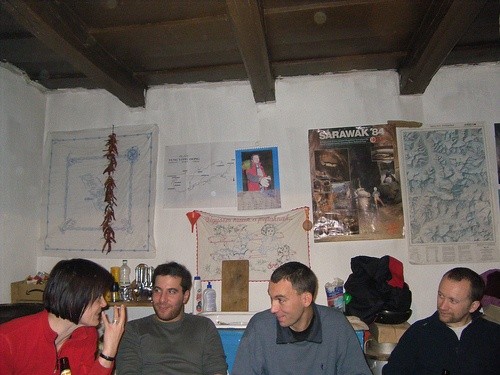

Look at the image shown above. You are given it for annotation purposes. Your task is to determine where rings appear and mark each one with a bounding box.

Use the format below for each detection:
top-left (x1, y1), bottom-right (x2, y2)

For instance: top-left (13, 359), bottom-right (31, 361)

top-left (113, 320), bottom-right (118, 323)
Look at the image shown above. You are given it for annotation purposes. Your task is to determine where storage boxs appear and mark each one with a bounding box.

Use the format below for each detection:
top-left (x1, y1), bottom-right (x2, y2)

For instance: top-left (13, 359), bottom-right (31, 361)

top-left (369, 322), bottom-right (411, 343)
top-left (10, 278), bottom-right (48, 305)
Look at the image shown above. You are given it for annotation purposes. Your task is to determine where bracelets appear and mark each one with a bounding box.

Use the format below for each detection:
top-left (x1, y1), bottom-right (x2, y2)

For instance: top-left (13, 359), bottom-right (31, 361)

top-left (100, 353), bottom-right (115, 361)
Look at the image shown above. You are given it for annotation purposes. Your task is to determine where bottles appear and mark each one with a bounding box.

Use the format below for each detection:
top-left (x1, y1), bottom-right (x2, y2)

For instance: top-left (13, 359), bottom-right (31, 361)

top-left (119, 260), bottom-right (131, 303)
top-left (203, 282), bottom-right (217, 312)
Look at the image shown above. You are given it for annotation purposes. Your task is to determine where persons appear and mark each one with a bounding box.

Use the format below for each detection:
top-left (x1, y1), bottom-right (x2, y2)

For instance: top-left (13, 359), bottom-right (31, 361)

top-left (0, 258), bottom-right (126, 375)
top-left (245, 154), bottom-right (271, 190)
top-left (230, 261), bottom-right (373, 375)
top-left (116, 262), bottom-right (229, 375)
top-left (382, 267), bottom-right (500, 375)
